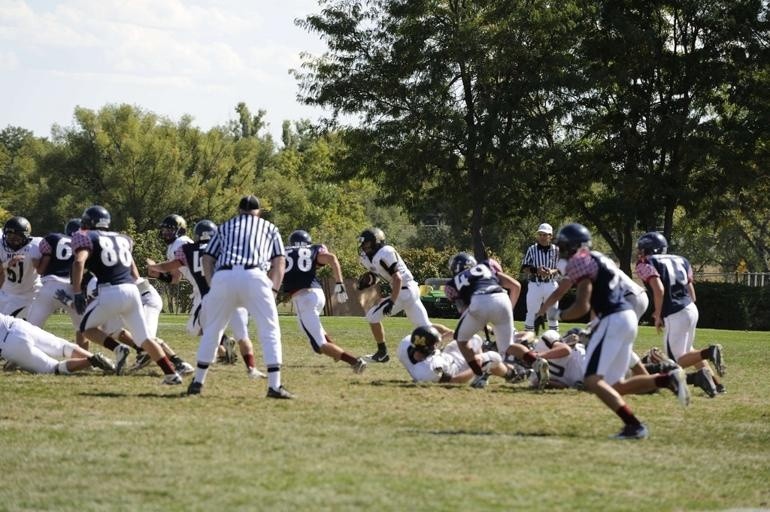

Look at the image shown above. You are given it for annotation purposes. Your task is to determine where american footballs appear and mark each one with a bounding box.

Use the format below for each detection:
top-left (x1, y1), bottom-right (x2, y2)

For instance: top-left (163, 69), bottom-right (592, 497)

top-left (362, 272), bottom-right (376, 285)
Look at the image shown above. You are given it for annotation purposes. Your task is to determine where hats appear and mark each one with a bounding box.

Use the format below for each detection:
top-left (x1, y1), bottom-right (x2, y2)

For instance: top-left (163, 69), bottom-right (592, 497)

top-left (536, 223), bottom-right (553, 235)
top-left (238, 195), bottom-right (261, 210)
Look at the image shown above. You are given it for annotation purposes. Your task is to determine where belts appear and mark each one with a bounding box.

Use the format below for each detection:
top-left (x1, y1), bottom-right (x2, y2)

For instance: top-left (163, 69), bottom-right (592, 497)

top-left (216, 265), bottom-right (257, 271)
top-left (530, 277), bottom-right (550, 283)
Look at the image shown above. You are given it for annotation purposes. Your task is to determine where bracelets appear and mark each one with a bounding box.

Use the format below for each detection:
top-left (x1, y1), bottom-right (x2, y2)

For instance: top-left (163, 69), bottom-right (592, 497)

top-left (271, 287), bottom-right (279, 294)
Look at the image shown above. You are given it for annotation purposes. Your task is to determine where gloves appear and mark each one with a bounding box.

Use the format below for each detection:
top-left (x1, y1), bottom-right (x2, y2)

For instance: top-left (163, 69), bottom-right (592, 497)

top-left (52, 289), bottom-right (74, 307)
top-left (333, 282), bottom-right (349, 304)
top-left (534, 303), bottom-right (549, 335)
top-left (546, 307), bottom-right (562, 321)
top-left (74, 290), bottom-right (88, 315)
top-left (383, 300), bottom-right (395, 315)
top-left (469, 372), bottom-right (489, 388)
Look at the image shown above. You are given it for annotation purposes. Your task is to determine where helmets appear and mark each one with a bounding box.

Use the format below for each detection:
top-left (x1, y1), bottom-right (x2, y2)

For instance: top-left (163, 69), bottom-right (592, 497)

top-left (288, 230), bottom-right (313, 244)
top-left (634, 232), bottom-right (669, 257)
top-left (79, 206), bottom-right (111, 230)
top-left (193, 220), bottom-right (218, 241)
top-left (554, 224), bottom-right (593, 259)
top-left (356, 227), bottom-right (386, 257)
top-left (65, 218), bottom-right (82, 236)
top-left (411, 325), bottom-right (443, 355)
top-left (448, 251), bottom-right (478, 277)
top-left (158, 214), bottom-right (188, 245)
top-left (2, 216), bottom-right (32, 252)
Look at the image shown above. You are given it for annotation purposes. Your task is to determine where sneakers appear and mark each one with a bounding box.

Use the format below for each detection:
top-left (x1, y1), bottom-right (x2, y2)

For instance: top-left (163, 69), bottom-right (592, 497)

top-left (247, 367), bottom-right (269, 379)
top-left (362, 350), bottom-right (389, 363)
top-left (531, 357), bottom-right (550, 393)
top-left (607, 423), bottom-right (646, 440)
top-left (86, 352), bottom-right (116, 375)
top-left (647, 348), bottom-right (669, 364)
top-left (716, 383), bottom-right (726, 393)
top-left (708, 344), bottom-right (726, 376)
top-left (128, 350), bottom-right (152, 372)
top-left (158, 370), bottom-right (184, 386)
top-left (113, 343), bottom-right (130, 376)
top-left (222, 336), bottom-right (236, 364)
top-left (188, 378), bottom-right (203, 395)
top-left (266, 384), bottom-right (297, 400)
top-left (659, 358), bottom-right (681, 372)
top-left (504, 365), bottom-right (534, 384)
top-left (694, 368), bottom-right (717, 397)
top-left (173, 362), bottom-right (194, 374)
top-left (351, 357), bottom-right (367, 374)
top-left (667, 368), bottom-right (690, 406)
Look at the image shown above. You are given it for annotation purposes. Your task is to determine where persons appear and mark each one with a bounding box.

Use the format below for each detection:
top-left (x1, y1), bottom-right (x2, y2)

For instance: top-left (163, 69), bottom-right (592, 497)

top-left (524, 225), bottom-right (563, 335)
top-left (533, 223), bottom-right (694, 438)
top-left (188, 195), bottom-right (296, 401)
top-left (358, 226), bottom-right (438, 361)
top-left (636, 231), bottom-right (728, 373)
top-left (0, 204), bottom-right (268, 384)
top-left (443, 252), bottom-right (550, 392)
top-left (394, 327), bottom-right (723, 404)
top-left (279, 231), bottom-right (368, 374)
